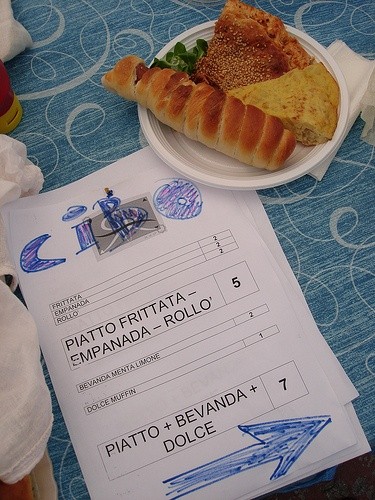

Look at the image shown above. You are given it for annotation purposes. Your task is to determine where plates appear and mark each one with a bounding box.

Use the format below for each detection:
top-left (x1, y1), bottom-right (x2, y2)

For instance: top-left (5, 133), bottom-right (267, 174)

top-left (137, 20), bottom-right (349, 191)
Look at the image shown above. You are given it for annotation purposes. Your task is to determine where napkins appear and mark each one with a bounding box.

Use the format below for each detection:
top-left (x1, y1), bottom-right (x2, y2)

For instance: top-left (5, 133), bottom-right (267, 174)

top-left (305, 38), bottom-right (375, 181)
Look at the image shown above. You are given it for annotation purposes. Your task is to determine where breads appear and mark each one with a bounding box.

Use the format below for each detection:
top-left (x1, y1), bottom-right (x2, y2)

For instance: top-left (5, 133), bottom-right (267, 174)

top-left (225, 61), bottom-right (341, 147)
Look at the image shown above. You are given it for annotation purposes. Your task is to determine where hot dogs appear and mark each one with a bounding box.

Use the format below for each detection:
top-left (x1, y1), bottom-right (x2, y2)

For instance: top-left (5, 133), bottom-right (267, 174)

top-left (100, 55), bottom-right (296, 171)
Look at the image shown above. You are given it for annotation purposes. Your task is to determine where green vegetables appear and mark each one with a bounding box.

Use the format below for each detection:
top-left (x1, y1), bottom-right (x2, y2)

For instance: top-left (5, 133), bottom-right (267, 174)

top-left (151, 38), bottom-right (209, 75)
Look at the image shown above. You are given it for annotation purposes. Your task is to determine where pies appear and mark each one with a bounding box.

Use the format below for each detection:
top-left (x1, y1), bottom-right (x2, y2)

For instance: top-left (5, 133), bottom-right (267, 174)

top-left (189, 0), bottom-right (316, 94)
